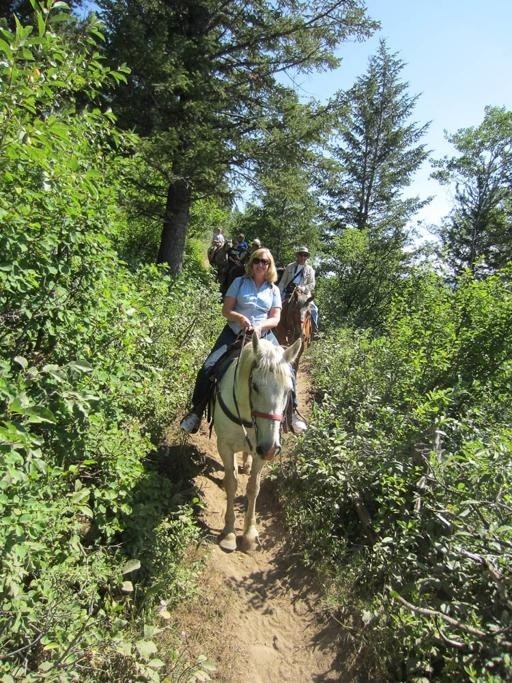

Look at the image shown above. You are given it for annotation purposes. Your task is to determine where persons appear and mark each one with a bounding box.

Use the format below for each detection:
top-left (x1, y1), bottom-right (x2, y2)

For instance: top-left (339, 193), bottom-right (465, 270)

top-left (277, 247), bottom-right (322, 339)
top-left (208, 227), bottom-right (262, 285)
top-left (180, 248), bottom-right (307, 434)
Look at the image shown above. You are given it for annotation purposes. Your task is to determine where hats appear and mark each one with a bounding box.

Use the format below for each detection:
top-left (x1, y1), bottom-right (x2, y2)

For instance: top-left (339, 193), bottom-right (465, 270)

top-left (295, 247), bottom-right (308, 253)
top-left (253, 237), bottom-right (261, 246)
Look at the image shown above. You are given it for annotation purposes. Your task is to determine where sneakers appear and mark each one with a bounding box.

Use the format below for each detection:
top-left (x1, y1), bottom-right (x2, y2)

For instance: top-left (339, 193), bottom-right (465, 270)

top-left (181, 413), bottom-right (201, 433)
top-left (292, 413), bottom-right (308, 434)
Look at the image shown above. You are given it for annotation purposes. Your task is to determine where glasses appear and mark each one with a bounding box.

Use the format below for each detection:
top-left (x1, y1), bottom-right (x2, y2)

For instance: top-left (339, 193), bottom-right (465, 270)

top-left (298, 253), bottom-right (308, 257)
top-left (253, 258), bottom-right (271, 265)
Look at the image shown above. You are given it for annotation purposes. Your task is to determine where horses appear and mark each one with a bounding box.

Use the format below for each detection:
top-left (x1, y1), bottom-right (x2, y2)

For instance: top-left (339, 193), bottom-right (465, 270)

top-left (206, 233), bottom-right (316, 553)
